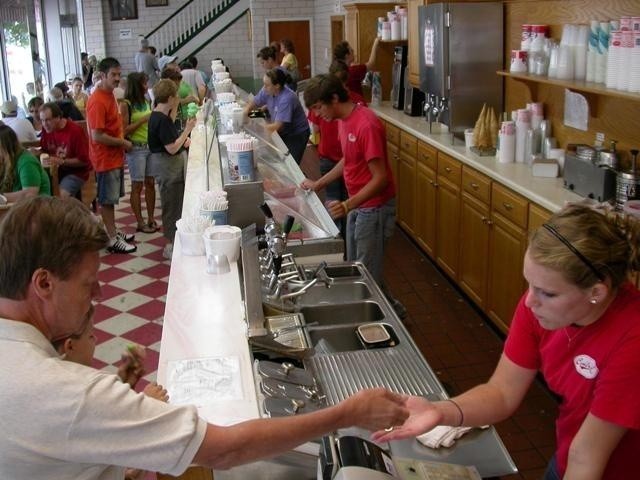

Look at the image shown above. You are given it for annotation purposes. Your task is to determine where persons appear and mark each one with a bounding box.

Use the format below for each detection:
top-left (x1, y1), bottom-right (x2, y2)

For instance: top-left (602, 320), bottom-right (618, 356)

top-left (0, 38), bottom-right (211, 256)
top-left (217, 57), bottom-right (240, 87)
top-left (50, 304), bottom-right (170, 480)
top-left (1, 196), bottom-right (410, 480)
top-left (366, 203), bottom-right (640, 480)
top-left (241, 36), bottom-right (408, 323)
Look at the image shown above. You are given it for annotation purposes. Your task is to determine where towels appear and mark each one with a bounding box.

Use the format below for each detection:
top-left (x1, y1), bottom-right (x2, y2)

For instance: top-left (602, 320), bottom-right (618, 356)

top-left (416, 424), bottom-right (490, 449)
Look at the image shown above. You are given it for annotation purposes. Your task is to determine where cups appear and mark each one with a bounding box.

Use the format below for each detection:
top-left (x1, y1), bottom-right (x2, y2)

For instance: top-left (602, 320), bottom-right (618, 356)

top-left (510, 17), bottom-right (640, 94)
top-left (40, 153), bottom-right (50, 168)
top-left (499, 102), bottom-right (558, 164)
top-left (376, 5), bottom-right (408, 41)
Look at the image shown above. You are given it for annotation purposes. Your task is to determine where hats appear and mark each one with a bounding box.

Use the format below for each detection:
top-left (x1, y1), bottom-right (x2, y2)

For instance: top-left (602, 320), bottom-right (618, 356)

top-left (0, 101), bottom-right (17, 115)
top-left (157, 55), bottom-right (177, 68)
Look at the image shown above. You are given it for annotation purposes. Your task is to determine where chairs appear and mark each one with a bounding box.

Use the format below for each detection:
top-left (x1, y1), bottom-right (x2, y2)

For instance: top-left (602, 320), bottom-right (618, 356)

top-left (23, 141), bottom-right (43, 149)
top-left (74, 120), bottom-right (100, 212)
top-left (44, 162), bottom-right (61, 196)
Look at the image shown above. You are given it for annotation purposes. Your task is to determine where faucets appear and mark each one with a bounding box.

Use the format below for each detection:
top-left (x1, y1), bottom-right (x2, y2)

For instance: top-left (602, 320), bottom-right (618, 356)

top-left (424, 94), bottom-right (435, 121)
top-left (431, 97), bottom-right (449, 125)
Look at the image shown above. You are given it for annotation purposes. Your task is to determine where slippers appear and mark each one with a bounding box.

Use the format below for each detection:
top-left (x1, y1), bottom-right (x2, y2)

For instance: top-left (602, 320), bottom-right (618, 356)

top-left (137, 221), bottom-right (160, 233)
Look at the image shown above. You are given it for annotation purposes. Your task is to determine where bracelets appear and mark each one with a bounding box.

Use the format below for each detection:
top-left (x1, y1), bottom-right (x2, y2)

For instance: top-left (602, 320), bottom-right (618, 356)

top-left (447, 398), bottom-right (465, 429)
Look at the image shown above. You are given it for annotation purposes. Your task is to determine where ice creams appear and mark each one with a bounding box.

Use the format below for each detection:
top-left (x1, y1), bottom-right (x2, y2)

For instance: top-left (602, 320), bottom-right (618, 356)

top-left (187, 103), bottom-right (198, 121)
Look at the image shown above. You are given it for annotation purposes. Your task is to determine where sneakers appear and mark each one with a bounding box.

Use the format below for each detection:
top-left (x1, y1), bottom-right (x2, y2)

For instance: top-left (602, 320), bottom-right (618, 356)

top-left (106, 232), bottom-right (137, 253)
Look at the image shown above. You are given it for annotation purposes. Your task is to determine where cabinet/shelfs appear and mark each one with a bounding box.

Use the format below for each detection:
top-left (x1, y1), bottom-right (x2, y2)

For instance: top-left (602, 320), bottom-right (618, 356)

top-left (384, 120), bottom-right (418, 239)
top-left (527, 202), bottom-right (552, 246)
top-left (417, 139), bottom-right (462, 285)
top-left (407, 0), bottom-right (449, 89)
top-left (458, 165), bottom-right (529, 336)
top-left (342, 2), bottom-right (408, 103)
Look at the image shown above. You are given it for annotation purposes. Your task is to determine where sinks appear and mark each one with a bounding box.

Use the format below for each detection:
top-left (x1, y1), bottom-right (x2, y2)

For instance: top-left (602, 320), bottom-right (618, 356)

top-left (299, 301), bottom-right (385, 326)
top-left (292, 282), bottom-right (373, 306)
top-left (304, 264), bottom-right (364, 283)
top-left (308, 322), bottom-right (401, 354)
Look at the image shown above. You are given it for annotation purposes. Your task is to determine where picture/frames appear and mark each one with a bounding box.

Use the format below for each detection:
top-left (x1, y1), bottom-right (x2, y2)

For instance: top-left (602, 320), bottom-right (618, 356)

top-left (145, 0), bottom-right (168, 7)
top-left (108, 0), bottom-right (138, 20)
